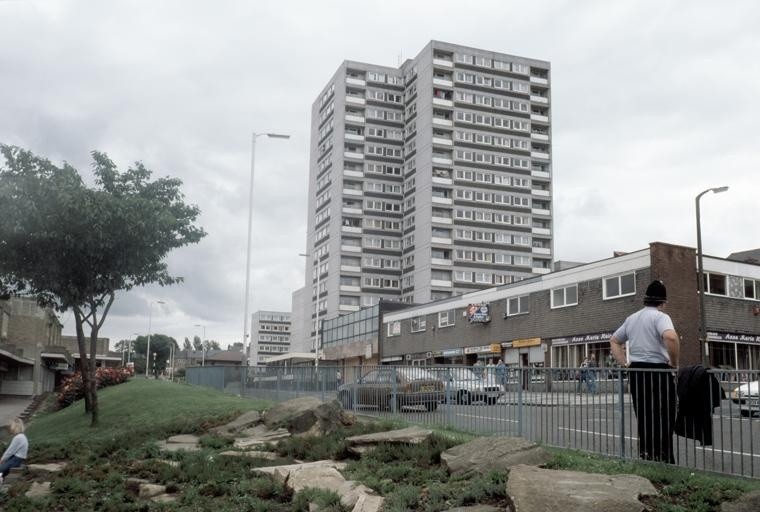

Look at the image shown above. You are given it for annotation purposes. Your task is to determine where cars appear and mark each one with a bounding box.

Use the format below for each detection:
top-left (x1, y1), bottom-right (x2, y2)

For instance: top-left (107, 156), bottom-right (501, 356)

top-left (429, 368), bottom-right (506, 406)
top-left (728, 381), bottom-right (760, 418)
top-left (336, 366), bottom-right (446, 413)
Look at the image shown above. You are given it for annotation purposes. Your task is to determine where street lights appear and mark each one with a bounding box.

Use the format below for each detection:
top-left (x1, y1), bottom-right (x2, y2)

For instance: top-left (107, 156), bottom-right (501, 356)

top-left (695, 184), bottom-right (728, 370)
top-left (127, 333), bottom-right (138, 363)
top-left (191, 324), bottom-right (205, 367)
top-left (145, 301), bottom-right (165, 379)
top-left (300, 253), bottom-right (321, 386)
top-left (242, 133), bottom-right (291, 390)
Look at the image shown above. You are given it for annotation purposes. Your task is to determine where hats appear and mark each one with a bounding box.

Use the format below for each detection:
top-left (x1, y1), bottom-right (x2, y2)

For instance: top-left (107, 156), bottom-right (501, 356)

top-left (641, 280), bottom-right (668, 302)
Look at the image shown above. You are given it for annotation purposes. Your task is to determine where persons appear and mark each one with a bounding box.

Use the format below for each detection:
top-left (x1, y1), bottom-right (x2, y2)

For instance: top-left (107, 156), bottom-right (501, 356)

top-left (0, 416), bottom-right (28, 473)
top-left (576, 357), bottom-right (590, 395)
top-left (587, 353), bottom-right (599, 394)
top-left (336, 369), bottom-right (342, 388)
top-left (609, 279), bottom-right (681, 464)
top-left (473, 357), bottom-right (508, 397)
